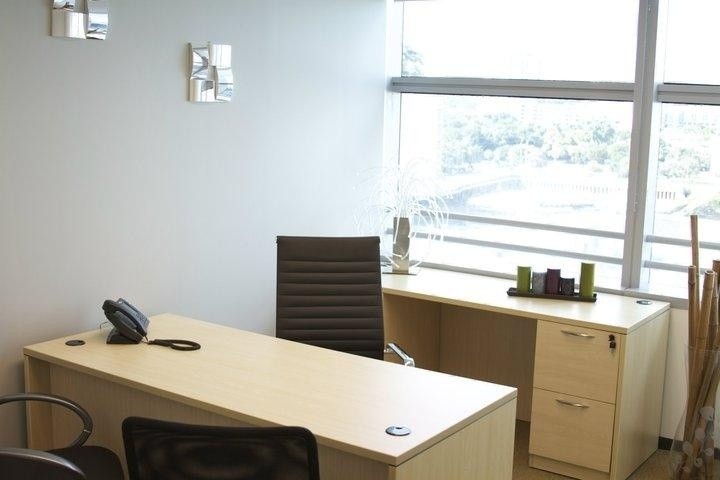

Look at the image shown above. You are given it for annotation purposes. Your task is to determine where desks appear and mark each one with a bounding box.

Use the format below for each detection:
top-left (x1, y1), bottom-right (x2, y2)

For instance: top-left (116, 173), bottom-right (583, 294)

top-left (17, 312), bottom-right (520, 480)
top-left (382, 261), bottom-right (673, 480)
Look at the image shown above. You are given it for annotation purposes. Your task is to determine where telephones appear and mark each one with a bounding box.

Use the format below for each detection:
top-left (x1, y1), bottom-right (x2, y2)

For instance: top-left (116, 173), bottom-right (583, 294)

top-left (102, 298), bottom-right (150, 344)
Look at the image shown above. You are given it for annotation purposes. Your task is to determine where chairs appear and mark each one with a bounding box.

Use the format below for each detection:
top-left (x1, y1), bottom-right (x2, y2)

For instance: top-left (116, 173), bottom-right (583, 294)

top-left (273, 234), bottom-right (415, 366)
top-left (121, 416), bottom-right (321, 479)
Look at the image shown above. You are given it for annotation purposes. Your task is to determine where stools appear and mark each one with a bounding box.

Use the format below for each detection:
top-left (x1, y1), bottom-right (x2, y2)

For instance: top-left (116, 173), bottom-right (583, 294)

top-left (1, 394), bottom-right (124, 479)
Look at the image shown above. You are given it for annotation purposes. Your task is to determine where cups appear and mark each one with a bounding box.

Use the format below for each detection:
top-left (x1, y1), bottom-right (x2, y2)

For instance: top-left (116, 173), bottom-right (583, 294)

top-left (516, 261), bottom-right (595, 296)
